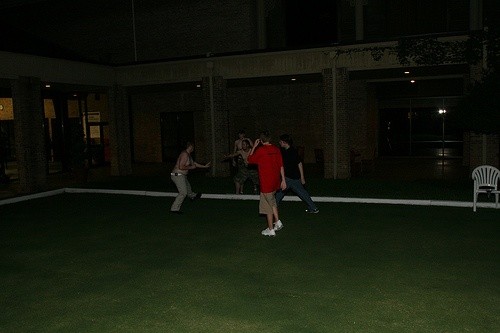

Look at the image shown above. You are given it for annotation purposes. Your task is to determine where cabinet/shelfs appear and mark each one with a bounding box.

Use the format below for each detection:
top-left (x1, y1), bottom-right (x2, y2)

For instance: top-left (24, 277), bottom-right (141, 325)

top-left (464, 134), bottom-right (499, 169)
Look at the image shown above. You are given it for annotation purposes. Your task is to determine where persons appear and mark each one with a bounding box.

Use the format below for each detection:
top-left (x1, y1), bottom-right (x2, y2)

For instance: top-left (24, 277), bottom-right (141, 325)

top-left (275, 134), bottom-right (320, 214)
top-left (225, 130), bottom-right (261, 195)
top-left (168, 141), bottom-right (213, 215)
top-left (244, 130), bottom-right (287, 236)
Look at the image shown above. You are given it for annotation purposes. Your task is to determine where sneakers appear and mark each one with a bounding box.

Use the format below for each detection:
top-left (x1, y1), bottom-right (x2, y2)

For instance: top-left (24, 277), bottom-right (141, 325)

top-left (169, 210), bottom-right (183, 216)
top-left (274, 220), bottom-right (284, 232)
top-left (261, 226), bottom-right (276, 236)
top-left (305, 209), bottom-right (319, 214)
top-left (189, 192), bottom-right (201, 201)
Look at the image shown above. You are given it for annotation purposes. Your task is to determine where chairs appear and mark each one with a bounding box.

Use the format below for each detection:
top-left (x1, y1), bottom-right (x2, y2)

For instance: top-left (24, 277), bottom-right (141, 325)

top-left (315, 148), bottom-right (324, 170)
top-left (472, 165), bottom-right (500, 212)
top-left (352, 150), bottom-right (371, 176)
